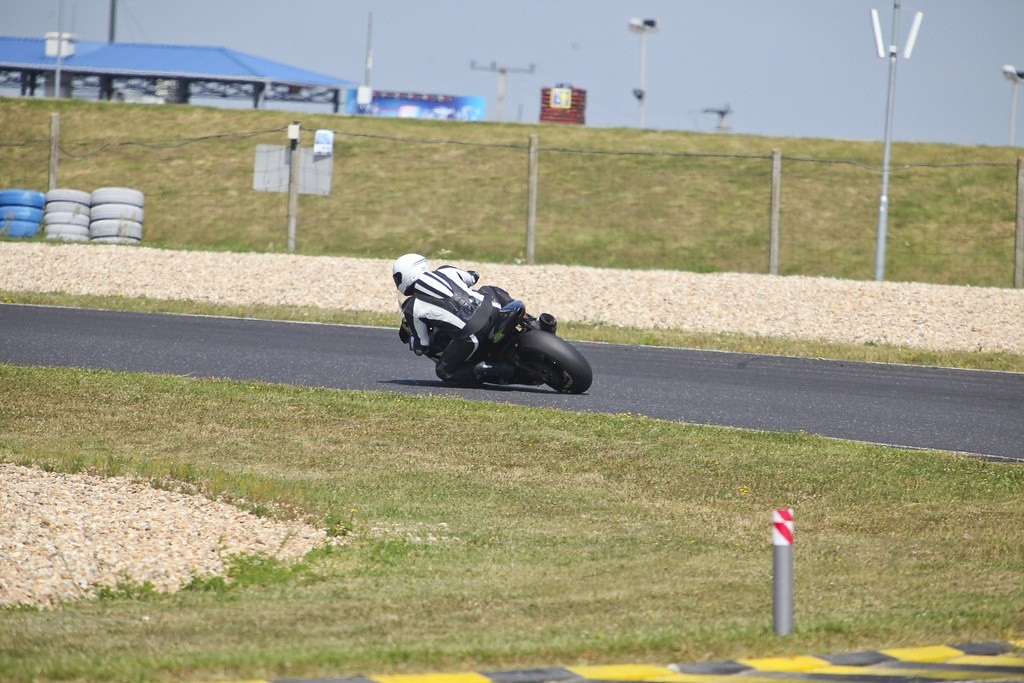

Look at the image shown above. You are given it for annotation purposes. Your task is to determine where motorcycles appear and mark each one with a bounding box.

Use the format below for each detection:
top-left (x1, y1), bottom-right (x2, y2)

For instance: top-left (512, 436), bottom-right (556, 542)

top-left (399, 300), bottom-right (593, 395)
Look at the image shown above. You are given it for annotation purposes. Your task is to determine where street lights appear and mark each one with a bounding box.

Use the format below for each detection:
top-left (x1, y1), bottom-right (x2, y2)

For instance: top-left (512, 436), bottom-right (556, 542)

top-left (999, 65), bottom-right (1023, 148)
top-left (630, 17), bottom-right (658, 125)
top-left (870, 9), bottom-right (924, 283)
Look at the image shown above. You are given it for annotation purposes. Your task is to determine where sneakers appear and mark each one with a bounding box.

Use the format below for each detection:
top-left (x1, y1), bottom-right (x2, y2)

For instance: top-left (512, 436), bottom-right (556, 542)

top-left (460, 364), bottom-right (484, 388)
top-left (521, 313), bottom-right (534, 323)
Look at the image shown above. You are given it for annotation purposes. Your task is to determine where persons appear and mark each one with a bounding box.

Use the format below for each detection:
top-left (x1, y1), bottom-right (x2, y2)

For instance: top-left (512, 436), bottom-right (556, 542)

top-left (393, 253), bottom-right (537, 388)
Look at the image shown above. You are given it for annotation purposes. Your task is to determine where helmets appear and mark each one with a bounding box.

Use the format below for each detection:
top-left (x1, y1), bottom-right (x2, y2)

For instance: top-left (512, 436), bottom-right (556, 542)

top-left (392, 252), bottom-right (429, 296)
